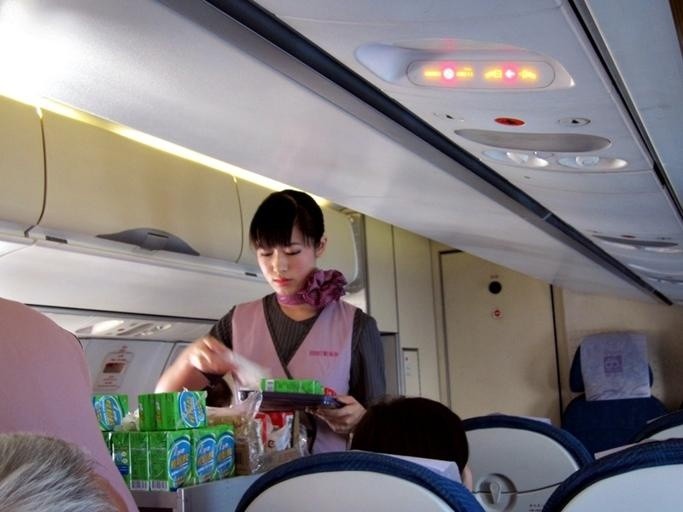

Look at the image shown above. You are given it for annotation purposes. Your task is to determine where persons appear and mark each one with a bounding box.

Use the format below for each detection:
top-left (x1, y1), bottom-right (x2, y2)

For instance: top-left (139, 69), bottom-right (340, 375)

top-left (155, 189), bottom-right (393, 468)
top-left (354, 392), bottom-right (472, 491)
top-left (0, 429), bottom-right (128, 512)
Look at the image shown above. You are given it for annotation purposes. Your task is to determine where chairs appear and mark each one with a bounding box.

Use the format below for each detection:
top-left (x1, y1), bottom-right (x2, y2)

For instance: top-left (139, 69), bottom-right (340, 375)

top-left (542, 438), bottom-right (683, 512)
top-left (562, 334), bottom-right (670, 451)
top-left (637, 411), bottom-right (682, 440)
top-left (462, 414), bottom-right (592, 512)
top-left (236, 451), bottom-right (485, 512)
